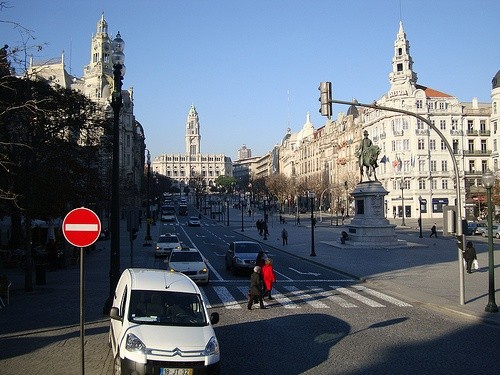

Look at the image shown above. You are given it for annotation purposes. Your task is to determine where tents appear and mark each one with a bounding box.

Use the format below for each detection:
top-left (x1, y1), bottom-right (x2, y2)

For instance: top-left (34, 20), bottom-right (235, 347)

top-left (0, 214), bottom-right (62, 240)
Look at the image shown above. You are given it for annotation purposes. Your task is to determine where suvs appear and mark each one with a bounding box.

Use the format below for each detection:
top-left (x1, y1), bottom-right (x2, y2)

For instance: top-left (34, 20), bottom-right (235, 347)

top-left (467, 222), bottom-right (487, 235)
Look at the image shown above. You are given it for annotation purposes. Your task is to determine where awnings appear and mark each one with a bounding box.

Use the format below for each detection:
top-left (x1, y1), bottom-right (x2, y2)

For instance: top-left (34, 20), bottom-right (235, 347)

top-left (472, 197), bottom-right (493, 201)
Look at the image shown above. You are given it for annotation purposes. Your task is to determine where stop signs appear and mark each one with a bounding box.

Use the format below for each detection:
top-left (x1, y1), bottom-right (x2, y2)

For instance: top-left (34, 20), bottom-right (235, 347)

top-left (61, 206), bottom-right (102, 248)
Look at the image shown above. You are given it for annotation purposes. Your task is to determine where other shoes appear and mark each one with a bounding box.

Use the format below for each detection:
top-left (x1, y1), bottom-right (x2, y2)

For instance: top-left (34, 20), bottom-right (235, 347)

top-left (260, 306), bottom-right (266, 309)
top-left (467, 271), bottom-right (472, 274)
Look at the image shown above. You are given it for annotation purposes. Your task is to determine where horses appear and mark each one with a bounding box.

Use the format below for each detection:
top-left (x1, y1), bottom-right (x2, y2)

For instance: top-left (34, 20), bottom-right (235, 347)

top-left (356, 145), bottom-right (382, 182)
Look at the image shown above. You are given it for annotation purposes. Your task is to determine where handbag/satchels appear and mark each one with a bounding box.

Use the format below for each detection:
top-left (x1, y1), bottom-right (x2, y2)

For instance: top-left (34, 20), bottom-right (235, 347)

top-left (473, 259), bottom-right (478, 269)
top-left (463, 258), bottom-right (465, 267)
top-left (246, 290), bottom-right (251, 298)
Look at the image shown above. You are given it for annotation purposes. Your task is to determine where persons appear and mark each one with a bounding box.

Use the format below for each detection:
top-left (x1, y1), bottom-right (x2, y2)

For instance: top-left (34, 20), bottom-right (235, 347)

top-left (248, 209), bottom-right (251, 217)
top-left (340, 231), bottom-right (348, 244)
top-left (280, 215), bottom-right (287, 224)
top-left (357, 130), bottom-right (379, 174)
top-left (464, 241), bottom-right (478, 273)
top-left (297, 216), bottom-right (300, 224)
top-left (429, 223), bottom-right (438, 238)
top-left (103, 228), bottom-right (109, 240)
top-left (281, 228), bottom-right (288, 246)
top-left (0, 271), bottom-right (9, 295)
top-left (247, 251), bottom-right (276, 310)
top-left (313, 218), bottom-right (317, 228)
top-left (256, 213), bottom-right (270, 236)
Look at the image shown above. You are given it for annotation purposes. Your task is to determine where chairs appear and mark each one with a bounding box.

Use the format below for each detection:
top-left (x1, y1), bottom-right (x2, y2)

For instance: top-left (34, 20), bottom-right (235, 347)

top-left (147, 294), bottom-right (162, 314)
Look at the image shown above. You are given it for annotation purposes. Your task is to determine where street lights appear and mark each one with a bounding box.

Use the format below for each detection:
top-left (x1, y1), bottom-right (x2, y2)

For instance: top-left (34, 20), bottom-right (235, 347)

top-left (308, 190), bottom-right (316, 257)
top-left (240, 193), bottom-right (245, 233)
top-left (418, 195), bottom-right (424, 238)
top-left (399, 178), bottom-right (406, 226)
top-left (482, 167), bottom-right (499, 313)
top-left (263, 196), bottom-right (267, 221)
top-left (102, 29), bottom-right (127, 316)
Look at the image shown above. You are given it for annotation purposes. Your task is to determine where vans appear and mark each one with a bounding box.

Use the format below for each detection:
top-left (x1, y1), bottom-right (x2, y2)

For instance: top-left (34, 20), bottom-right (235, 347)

top-left (107, 267), bottom-right (221, 375)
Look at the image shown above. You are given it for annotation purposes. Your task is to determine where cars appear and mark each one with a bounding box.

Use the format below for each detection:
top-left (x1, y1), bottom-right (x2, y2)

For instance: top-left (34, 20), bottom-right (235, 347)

top-left (225, 241), bottom-right (269, 277)
top-left (188, 216), bottom-right (201, 227)
top-left (480, 225), bottom-right (500, 239)
top-left (160, 193), bottom-right (189, 222)
top-left (153, 233), bottom-right (184, 258)
top-left (164, 246), bottom-right (210, 285)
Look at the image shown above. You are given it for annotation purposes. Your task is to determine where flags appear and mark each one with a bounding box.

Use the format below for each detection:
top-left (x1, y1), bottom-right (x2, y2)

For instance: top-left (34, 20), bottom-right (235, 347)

top-left (393, 160), bottom-right (398, 168)
top-left (380, 155), bottom-right (386, 164)
